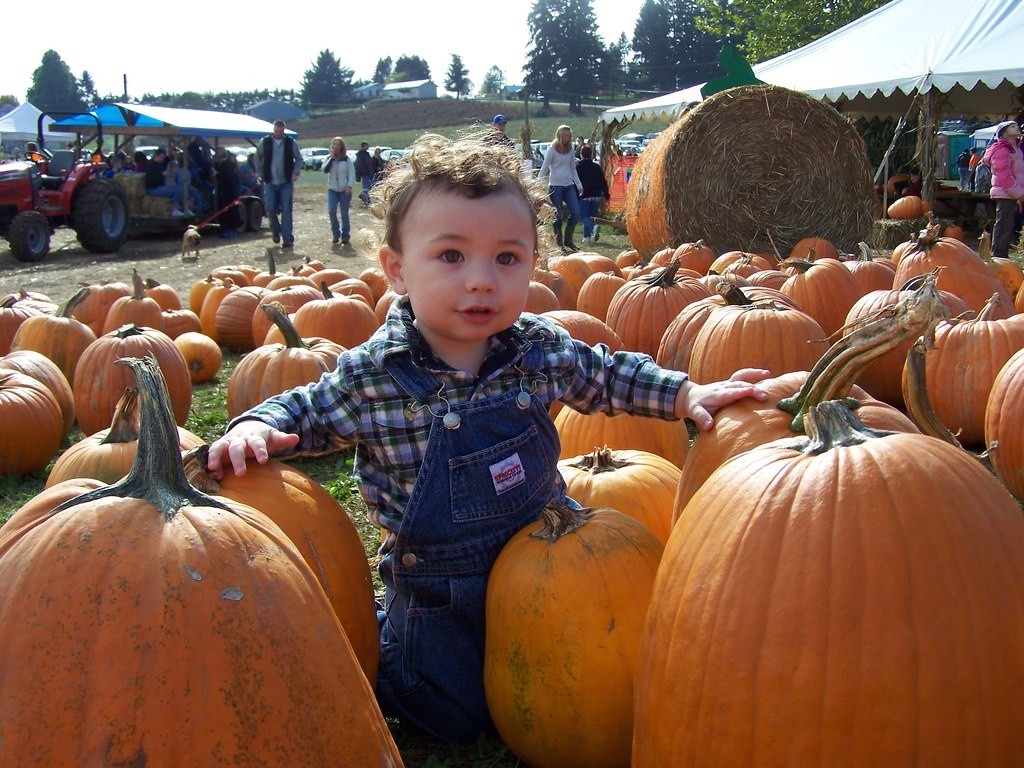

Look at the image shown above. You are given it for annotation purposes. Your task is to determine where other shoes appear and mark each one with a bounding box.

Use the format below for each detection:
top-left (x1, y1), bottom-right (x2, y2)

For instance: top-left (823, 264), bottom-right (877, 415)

top-left (171, 210), bottom-right (185, 216)
top-left (283, 241), bottom-right (293, 247)
top-left (333, 235), bottom-right (339, 242)
top-left (184, 210), bottom-right (195, 216)
top-left (273, 231), bottom-right (280, 242)
top-left (342, 238), bottom-right (349, 244)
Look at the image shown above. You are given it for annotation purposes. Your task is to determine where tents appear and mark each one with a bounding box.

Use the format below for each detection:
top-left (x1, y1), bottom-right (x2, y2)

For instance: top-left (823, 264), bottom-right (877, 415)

top-left (48, 102), bottom-right (299, 215)
top-left (0, 100), bottom-right (82, 146)
top-left (595, 0), bottom-right (1024, 202)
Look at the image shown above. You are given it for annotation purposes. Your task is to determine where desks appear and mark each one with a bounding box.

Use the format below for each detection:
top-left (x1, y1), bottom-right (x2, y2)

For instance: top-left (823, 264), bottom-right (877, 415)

top-left (877, 189), bottom-right (996, 234)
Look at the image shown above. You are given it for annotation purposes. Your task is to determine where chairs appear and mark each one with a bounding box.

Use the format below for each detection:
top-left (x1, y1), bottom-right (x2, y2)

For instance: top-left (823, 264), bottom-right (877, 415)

top-left (40, 151), bottom-right (73, 191)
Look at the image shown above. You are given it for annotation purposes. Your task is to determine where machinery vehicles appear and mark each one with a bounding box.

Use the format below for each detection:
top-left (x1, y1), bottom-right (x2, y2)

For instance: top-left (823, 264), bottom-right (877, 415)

top-left (0, 102), bottom-right (299, 262)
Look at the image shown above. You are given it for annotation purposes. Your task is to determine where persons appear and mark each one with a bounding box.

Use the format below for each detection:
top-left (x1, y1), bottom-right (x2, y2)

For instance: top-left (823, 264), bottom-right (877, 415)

top-left (483, 115), bottom-right (515, 149)
top-left (372, 146), bottom-right (389, 182)
top-left (209, 115), bottom-right (768, 768)
top-left (900, 165), bottom-right (922, 200)
top-left (8, 142), bottom-right (39, 161)
top-left (321, 136), bottom-right (355, 244)
top-left (611, 144), bottom-right (623, 156)
top-left (977, 149), bottom-right (985, 164)
top-left (536, 125), bottom-right (584, 252)
top-left (575, 146), bottom-right (610, 243)
top-left (214, 119), bottom-right (304, 248)
top-left (354, 141), bottom-right (375, 204)
top-left (955, 146), bottom-right (980, 192)
top-left (574, 136), bottom-right (586, 159)
top-left (625, 146), bottom-right (638, 182)
top-left (103, 135), bottom-right (215, 215)
top-left (984, 121), bottom-right (1024, 258)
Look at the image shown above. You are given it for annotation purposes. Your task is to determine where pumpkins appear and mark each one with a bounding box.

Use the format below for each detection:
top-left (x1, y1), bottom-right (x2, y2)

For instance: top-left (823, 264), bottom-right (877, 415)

top-left (485, 173), bottom-right (1024, 768)
top-left (0, 250), bottom-right (403, 768)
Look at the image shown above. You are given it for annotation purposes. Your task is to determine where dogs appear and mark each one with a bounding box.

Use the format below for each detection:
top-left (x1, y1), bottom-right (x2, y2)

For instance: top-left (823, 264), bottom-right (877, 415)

top-left (180, 225), bottom-right (202, 259)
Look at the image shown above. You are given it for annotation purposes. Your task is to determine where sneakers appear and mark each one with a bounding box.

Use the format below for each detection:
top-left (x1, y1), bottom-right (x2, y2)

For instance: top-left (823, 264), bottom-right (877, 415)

top-left (582, 236), bottom-right (590, 243)
top-left (592, 225), bottom-right (600, 241)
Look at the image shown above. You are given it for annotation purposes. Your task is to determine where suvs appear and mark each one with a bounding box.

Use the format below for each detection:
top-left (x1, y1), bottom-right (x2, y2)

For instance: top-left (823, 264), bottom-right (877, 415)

top-left (529, 93), bottom-right (544, 103)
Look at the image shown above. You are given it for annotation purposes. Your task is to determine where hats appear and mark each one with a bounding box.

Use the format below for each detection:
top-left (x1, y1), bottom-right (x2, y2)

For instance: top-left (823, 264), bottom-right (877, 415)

top-left (493, 114), bottom-right (507, 124)
top-left (997, 120), bottom-right (1017, 139)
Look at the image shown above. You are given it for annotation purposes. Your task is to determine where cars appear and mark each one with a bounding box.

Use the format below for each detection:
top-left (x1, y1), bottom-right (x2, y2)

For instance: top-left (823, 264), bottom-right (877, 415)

top-left (300, 147), bottom-right (422, 202)
top-left (511, 133), bottom-right (661, 197)
top-left (136, 145), bottom-right (258, 167)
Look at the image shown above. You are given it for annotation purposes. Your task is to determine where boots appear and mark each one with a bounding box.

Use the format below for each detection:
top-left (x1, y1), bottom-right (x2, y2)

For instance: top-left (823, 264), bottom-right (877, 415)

top-left (554, 229), bottom-right (566, 251)
top-left (564, 224), bottom-right (580, 251)
